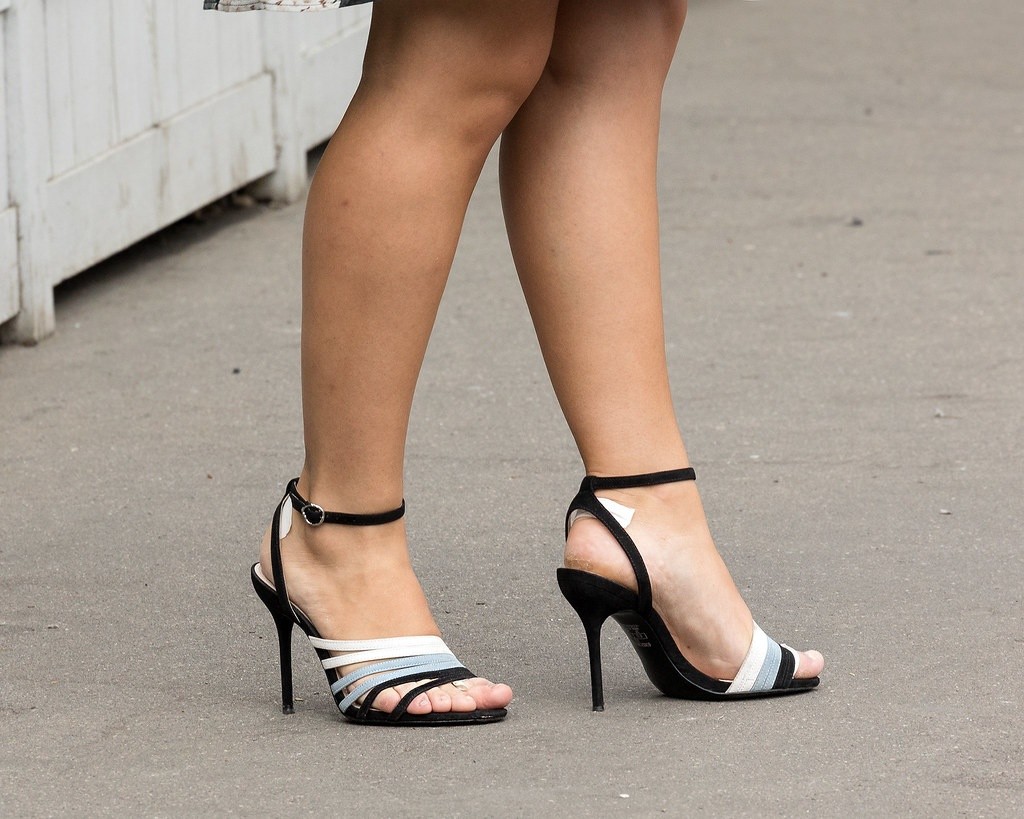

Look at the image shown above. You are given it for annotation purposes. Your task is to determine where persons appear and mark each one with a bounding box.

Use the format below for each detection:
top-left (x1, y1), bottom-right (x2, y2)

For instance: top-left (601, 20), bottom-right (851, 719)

top-left (204, 0), bottom-right (824, 726)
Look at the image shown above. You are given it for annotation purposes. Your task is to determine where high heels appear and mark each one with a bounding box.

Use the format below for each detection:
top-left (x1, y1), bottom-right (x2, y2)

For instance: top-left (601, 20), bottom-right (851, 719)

top-left (252, 477), bottom-right (507, 726)
top-left (555, 467), bottom-right (821, 712)
